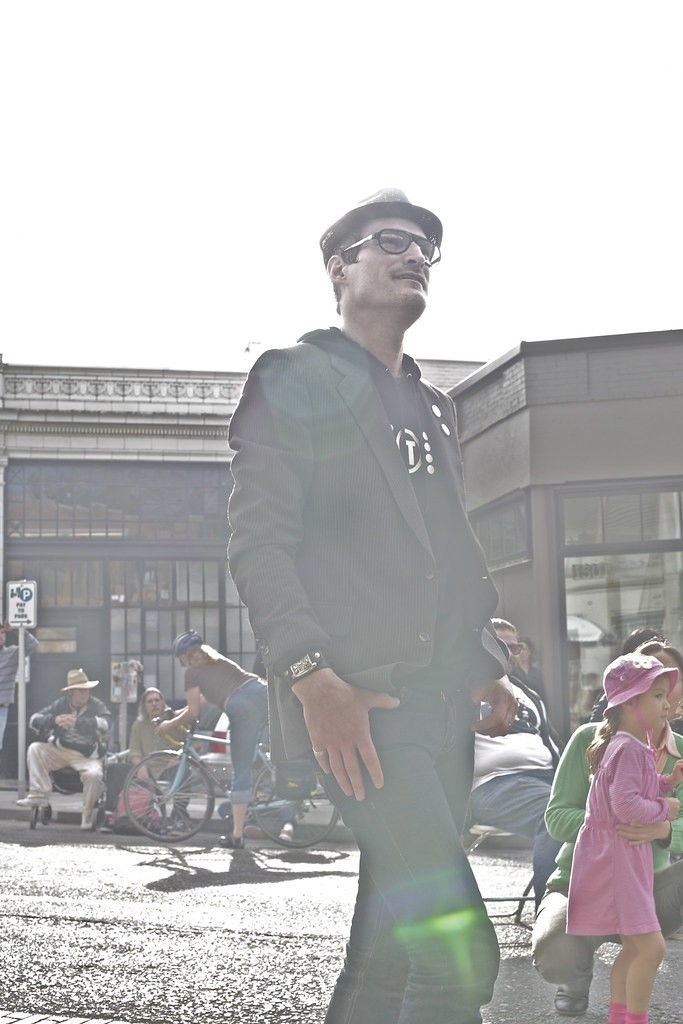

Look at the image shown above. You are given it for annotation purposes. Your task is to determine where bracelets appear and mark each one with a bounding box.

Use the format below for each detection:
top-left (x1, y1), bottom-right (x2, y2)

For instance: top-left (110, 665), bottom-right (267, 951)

top-left (282, 648), bottom-right (330, 686)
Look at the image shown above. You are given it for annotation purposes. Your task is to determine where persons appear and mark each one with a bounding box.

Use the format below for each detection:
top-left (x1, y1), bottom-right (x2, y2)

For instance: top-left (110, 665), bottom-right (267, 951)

top-left (228, 188), bottom-right (519, 1024)
top-left (528, 627), bottom-right (683, 1024)
top-left (0, 625), bottom-right (40, 749)
top-left (14, 668), bottom-right (113, 831)
top-left (564, 651), bottom-right (683, 1024)
top-left (127, 616), bottom-right (565, 912)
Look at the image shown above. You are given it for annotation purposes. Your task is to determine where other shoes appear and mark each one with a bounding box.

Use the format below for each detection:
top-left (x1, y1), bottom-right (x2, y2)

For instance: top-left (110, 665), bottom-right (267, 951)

top-left (15, 797), bottom-right (48, 808)
top-left (243, 825), bottom-right (269, 840)
top-left (82, 814), bottom-right (93, 830)
top-left (278, 828), bottom-right (294, 842)
top-left (554, 986), bottom-right (588, 1015)
top-left (217, 834), bottom-right (245, 849)
top-left (100, 811), bottom-right (116, 833)
top-left (171, 820), bottom-right (191, 836)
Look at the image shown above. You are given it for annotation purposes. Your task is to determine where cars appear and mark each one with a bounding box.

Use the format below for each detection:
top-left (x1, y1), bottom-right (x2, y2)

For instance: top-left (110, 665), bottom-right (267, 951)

top-left (208, 712), bottom-right (325, 797)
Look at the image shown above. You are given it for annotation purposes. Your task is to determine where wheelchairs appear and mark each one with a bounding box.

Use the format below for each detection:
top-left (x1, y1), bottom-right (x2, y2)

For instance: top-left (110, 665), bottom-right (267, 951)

top-left (30, 730), bottom-right (108, 830)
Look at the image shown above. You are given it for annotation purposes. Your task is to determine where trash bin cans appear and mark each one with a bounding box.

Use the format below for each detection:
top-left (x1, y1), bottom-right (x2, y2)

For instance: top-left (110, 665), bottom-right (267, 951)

top-left (106, 750), bottom-right (132, 812)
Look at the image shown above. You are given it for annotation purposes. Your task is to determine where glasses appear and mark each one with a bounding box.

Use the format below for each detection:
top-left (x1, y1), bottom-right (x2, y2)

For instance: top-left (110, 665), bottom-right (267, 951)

top-left (507, 643), bottom-right (524, 656)
top-left (339, 228), bottom-right (441, 267)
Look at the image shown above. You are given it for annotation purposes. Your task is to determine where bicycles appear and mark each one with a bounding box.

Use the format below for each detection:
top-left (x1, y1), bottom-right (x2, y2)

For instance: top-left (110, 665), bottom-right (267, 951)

top-left (122, 718), bottom-right (341, 849)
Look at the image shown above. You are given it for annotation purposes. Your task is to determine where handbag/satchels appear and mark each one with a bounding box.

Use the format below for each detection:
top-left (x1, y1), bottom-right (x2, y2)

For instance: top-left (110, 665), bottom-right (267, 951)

top-left (61, 730), bottom-right (97, 757)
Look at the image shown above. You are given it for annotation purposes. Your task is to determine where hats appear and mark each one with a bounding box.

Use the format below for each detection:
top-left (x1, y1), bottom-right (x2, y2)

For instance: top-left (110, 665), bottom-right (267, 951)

top-left (602, 652), bottom-right (679, 719)
top-left (60, 669), bottom-right (99, 692)
top-left (320, 187), bottom-right (443, 266)
top-left (173, 630), bottom-right (203, 668)
